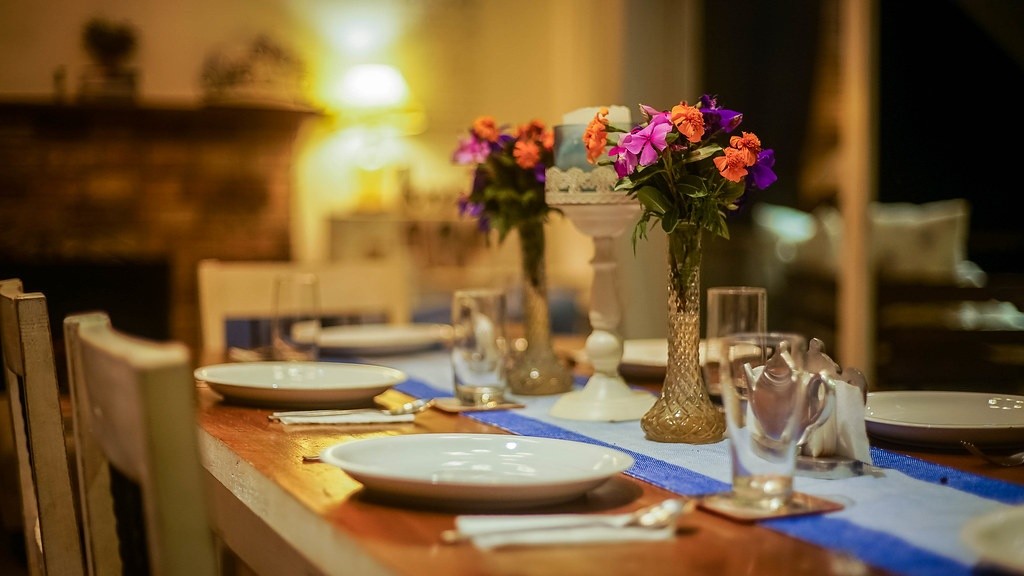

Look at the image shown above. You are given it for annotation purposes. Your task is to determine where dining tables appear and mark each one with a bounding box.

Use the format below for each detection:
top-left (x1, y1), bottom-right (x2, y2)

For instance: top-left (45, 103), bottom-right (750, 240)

top-left (196, 355), bottom-right (1024, 576)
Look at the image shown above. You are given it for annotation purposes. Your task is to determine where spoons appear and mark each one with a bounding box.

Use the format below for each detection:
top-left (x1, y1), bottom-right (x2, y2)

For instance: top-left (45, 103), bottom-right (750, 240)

top-left (442, 497), bottom-right (698, 545)
top-left (267, 398), bottom-right (436, 421)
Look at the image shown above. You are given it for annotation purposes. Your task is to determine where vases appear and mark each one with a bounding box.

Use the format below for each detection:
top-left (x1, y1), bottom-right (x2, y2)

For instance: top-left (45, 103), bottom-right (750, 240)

top-left (506, 220), bottom-right (573, 396)
top-left (639, 220), bottom-right (727, 444)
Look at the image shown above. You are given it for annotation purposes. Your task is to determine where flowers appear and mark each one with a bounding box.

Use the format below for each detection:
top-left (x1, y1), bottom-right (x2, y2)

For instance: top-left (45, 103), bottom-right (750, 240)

top-left (448, 113), bottom-right (564, 298)
top-left (584, 94), bottom-right (777, 314)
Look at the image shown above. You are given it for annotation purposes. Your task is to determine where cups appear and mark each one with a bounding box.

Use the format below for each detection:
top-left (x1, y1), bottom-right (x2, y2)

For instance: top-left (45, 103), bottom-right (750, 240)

top-left (452, 289), bottom-right (507, 407)
top-left (704, 287), bottom-right (767, 413)
top-left (719, 333), bottom-right (805, 514)
top-left (270, 275), bottom-right (321, 361)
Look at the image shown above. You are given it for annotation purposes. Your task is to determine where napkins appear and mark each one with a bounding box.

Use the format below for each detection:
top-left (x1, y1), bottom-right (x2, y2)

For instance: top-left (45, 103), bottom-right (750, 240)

top-left (453, 510), bottom-right (678, 550)
top-left (272, 410), bottom-right (415, 425)
top-left (753, 368), bottom-right (873, 467)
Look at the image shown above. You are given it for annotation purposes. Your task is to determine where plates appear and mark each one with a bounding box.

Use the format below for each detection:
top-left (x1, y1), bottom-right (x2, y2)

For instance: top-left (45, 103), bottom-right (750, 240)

top-left (573, 339), bottom-right (772, 380)
top-left (957, 504), bottom-right (1024, 576)
top-left (194, 361), bottom-right (409, 410)
top-left (320, 433), bottom-right (634, 511)
top-left (865, 391), bottom-right (1023, 449)
top-left (322, 323), bottom-right (450, 354)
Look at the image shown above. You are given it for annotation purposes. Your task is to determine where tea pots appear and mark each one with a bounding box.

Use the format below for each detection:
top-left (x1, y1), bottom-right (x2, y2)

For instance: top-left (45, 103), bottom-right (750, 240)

top-left (741, 342), bottom-right (834, 455)
top-left (741, 338), bottom-right (867, 457)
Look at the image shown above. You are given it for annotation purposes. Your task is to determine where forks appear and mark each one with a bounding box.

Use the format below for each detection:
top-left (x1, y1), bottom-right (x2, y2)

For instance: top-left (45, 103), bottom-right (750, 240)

top-left (960, 440), bottom-right (1023, 467)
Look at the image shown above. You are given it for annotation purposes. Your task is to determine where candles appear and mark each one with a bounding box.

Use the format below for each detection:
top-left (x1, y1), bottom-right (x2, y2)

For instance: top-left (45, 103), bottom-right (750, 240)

top-left (554, 103), bottom-right (632, 173)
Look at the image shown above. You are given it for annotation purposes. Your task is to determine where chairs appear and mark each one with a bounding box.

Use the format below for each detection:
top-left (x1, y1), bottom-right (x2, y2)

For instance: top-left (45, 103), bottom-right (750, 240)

top-left (64, 311), bottom-right (219, 576)
top-left (0, 278), bottom-right (82, 576)
top-left (872, 260), bottom-right (1024, 395)
top-left (196, 252), bottom-right (412, 368)
